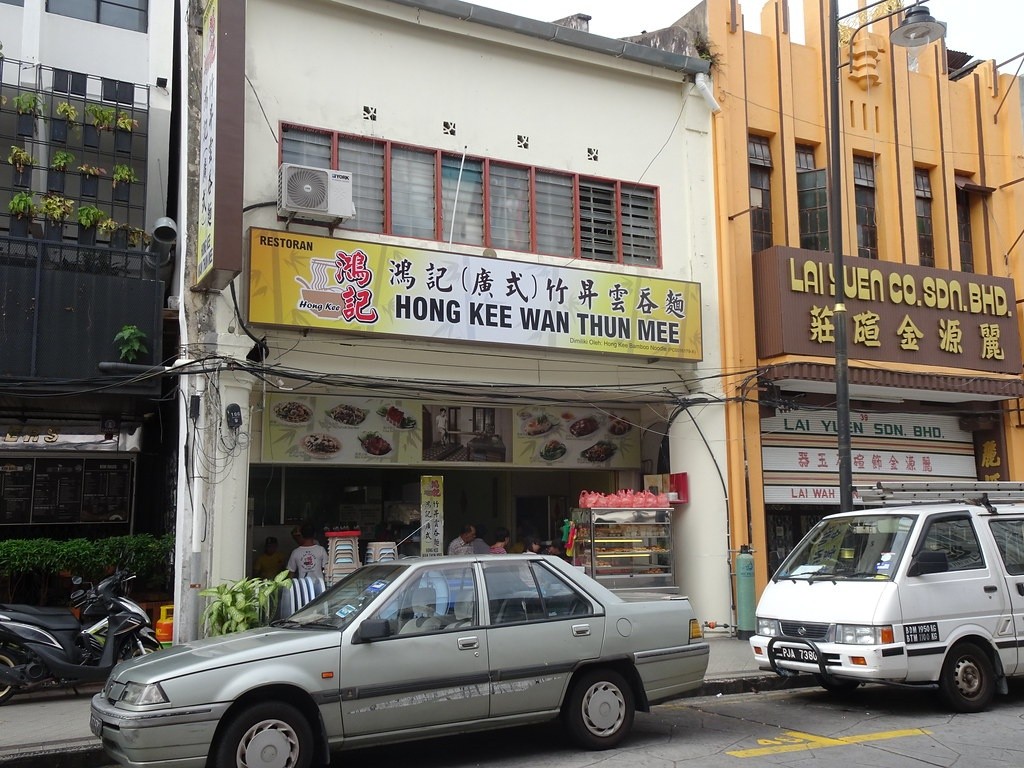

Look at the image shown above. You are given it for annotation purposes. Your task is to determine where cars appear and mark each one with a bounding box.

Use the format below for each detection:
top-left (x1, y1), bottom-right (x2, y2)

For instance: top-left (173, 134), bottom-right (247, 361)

top-left (90, 550), bottom-right (713, 768)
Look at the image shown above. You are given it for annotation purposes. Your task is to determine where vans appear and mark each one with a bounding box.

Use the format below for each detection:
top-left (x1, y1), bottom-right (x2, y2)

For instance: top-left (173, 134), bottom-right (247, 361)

top-left (748, 481), bottom-right (1024, 715)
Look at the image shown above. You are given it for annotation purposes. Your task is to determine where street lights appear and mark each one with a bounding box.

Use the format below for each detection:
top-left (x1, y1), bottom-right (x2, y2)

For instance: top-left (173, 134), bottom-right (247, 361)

top-left (825, 0), bottom-right (952, 512)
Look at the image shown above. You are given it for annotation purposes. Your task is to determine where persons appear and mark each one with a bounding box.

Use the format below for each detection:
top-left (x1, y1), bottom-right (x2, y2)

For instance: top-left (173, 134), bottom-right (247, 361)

top-left (286, 524), bottom-right (328, 594)
top-left (290, 525), bottom-right (320, 545)
top-left (447, 525), bottom-right (477, 577)
top-left (472, 523), bottom-right (490, 554)
top-left (487, 527), bottom-right (511, 554)
top-left (254, 537), bottom-right (294, 578)
top-left (521, 534), bottom-right (570, 613)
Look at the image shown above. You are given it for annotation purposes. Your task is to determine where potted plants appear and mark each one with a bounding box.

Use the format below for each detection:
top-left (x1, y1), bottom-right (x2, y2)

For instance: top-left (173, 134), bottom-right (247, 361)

top-left (48, 150), bottom-right (75, 193)
top-left (0, 533), bottom-right (175, 578)
top-left (75, 204), bottom-right (111, 245)
top-left (49, 101), bottom-right (82, 143)
top-left (77, 164), bottom-right (107, 197)
top-left (38, 191), bottom-right (76, 241)
top-left (8, 192), bottom-right (40, 237)
top-left (7, 145), bottom-right (38, 187)
top-left (83, 104), bottom-right (116, 148)
top-left (113, 111), bottom-right (139, 152)
top-left (97, 218), bottom-right (151, 250)
top-left (112, 162), bottom-right (140, 202)
top-left (13, 93), bottom-right (49, 138)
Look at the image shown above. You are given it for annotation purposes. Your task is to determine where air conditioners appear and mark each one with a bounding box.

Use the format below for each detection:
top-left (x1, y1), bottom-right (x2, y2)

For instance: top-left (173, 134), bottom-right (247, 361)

top-left (274, 162), bottom-right (354, 223)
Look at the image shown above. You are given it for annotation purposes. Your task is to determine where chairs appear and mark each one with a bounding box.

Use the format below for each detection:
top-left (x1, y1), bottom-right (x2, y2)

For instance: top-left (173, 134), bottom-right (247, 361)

top-left (398, 587), bottom-right (499, 636)
top-left (496, 591), bottom-right (548, 623)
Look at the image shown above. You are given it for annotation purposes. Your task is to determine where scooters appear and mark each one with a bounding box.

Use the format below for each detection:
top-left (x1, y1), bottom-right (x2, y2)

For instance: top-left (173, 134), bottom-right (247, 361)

top-left (0, 549), bottom-right (167, 706)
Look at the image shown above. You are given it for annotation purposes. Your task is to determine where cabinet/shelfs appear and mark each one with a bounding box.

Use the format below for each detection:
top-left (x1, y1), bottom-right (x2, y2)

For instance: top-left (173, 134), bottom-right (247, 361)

top-left (569, 507), bottom-right (676, 586)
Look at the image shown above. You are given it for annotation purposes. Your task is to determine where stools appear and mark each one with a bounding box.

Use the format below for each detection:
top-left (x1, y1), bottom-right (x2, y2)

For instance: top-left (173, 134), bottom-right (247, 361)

top-left (323, 531), bottom-right (399, 614)
